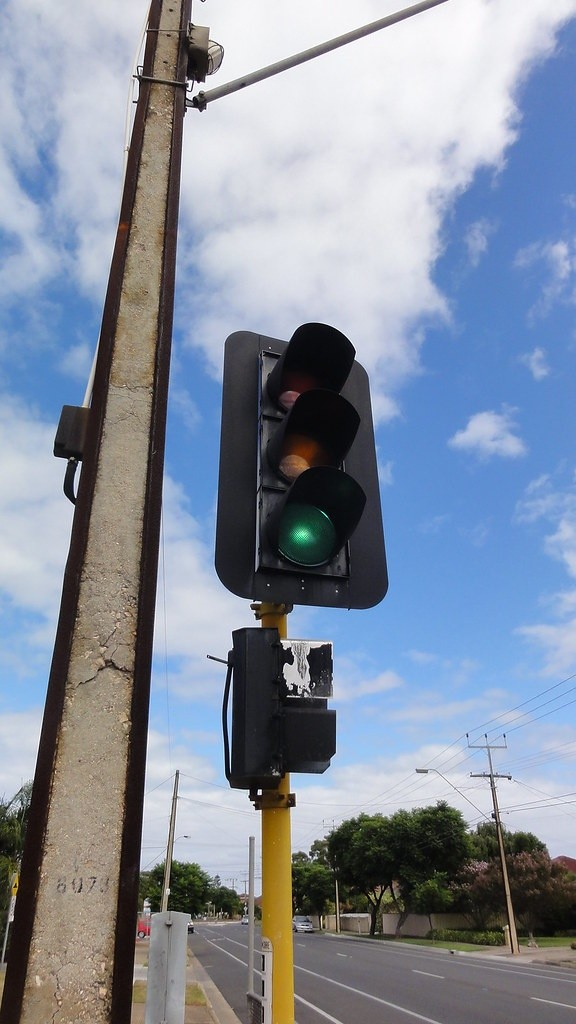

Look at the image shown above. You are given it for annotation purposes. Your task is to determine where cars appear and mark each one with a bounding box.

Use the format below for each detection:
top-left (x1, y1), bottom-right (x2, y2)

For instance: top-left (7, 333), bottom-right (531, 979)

top-left (241, 915), bottom-right (249, 925)
top-left (188, 920), bottom-right (194, 933)
top-left (292, 915), bottom-right (313, 933)
top-left (136, 918), bottom-right (152, 939)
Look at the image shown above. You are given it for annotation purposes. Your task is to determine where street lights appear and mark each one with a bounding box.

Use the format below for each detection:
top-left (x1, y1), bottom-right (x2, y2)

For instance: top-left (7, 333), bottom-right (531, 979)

top-left (413, 767), bottom-right (521, 957)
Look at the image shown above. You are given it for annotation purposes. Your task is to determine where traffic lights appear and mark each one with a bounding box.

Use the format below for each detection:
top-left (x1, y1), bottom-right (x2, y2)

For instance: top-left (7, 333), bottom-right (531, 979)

top-left (214, 322), bottom-right (389, 611)
